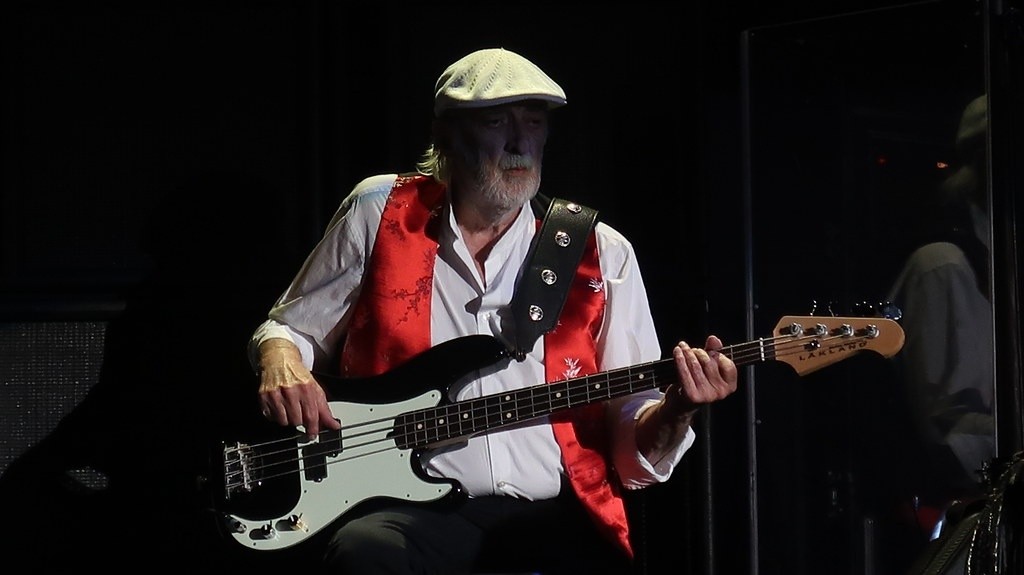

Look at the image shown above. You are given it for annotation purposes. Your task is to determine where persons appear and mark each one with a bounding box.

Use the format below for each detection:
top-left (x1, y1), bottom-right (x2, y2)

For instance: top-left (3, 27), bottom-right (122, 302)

top-left (885, 92), bottom-right (998, 572)
top-left (245, 46), bottom-right (738, 575)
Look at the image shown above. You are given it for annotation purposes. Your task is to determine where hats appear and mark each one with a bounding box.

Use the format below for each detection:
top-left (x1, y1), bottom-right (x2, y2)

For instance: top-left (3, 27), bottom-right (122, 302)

top-left (433, 47), bottom-right (568, 117)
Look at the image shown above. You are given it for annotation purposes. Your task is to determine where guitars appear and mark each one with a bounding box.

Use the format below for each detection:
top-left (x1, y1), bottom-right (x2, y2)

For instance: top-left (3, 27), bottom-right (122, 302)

top-left (184, 300), bottom-right (907, 569)
